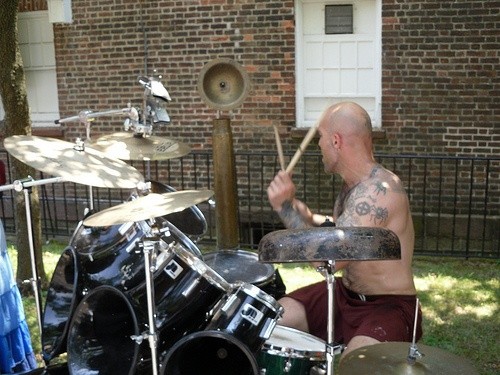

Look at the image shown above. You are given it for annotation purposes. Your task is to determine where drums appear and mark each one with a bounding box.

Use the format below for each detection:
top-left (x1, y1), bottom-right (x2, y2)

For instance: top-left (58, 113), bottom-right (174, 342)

top-left (208, 281), bottom-right (289, 355)
top-left (196, 250), bottom-right (290, 303)
top-left (148, 248), bottom-right (232, 358)
top-left (102, 217), bottom-right (203, 293)
top-left (260, 324), bottom-right (329, 375)
top-left (121, 176), bottom-right (207, 240)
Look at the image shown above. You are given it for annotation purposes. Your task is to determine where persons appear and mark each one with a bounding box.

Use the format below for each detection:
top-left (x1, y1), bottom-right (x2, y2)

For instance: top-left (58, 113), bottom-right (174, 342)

top-left (266, 101), bottom-right (422, 367)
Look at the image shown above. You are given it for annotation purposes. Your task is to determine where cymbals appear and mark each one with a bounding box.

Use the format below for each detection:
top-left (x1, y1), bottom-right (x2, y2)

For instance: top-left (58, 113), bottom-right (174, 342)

top-left (2, 134), bottom-right (147, 192)
top-left (336, 343), bottom-right (488, 375)
top-left (254, 221), bottom-right (402, 264)
top-left (80, 187), bottom-right (214, 228)
top-left (81, 124), bottom-right (191, 161)
